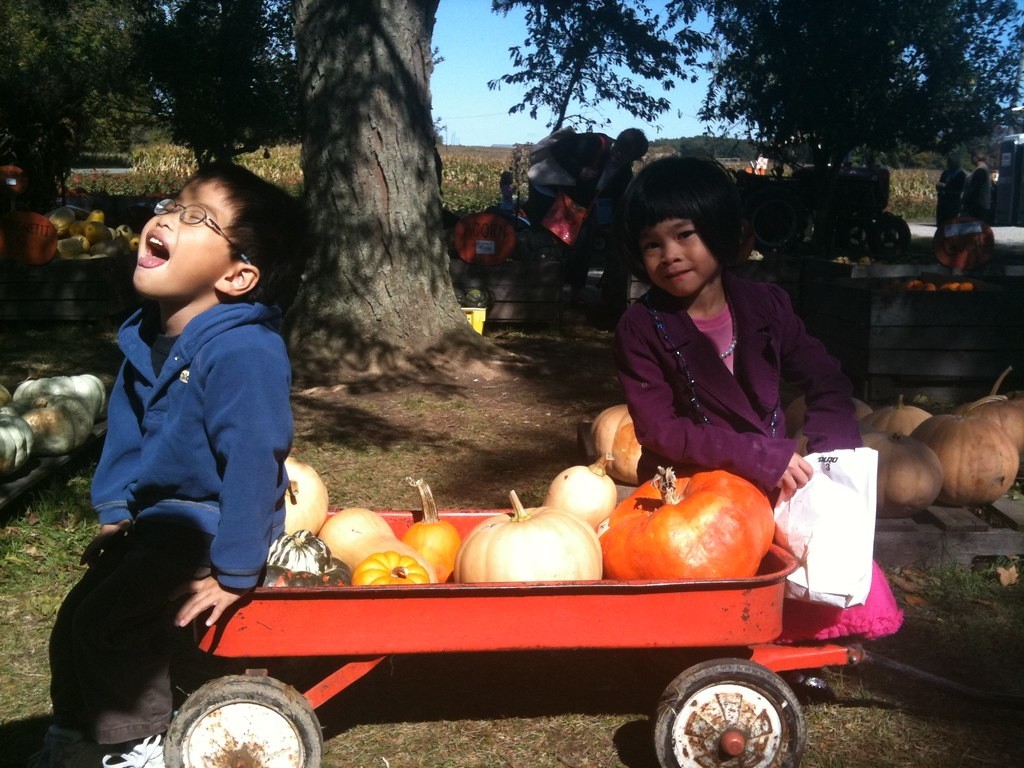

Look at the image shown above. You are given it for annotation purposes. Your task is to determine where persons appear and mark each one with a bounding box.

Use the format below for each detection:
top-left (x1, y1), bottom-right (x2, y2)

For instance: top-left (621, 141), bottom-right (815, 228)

top-left (962, 150), bottom-right (992, 220)
top-left (34, 165), bottom-right (309, 767)
top-left (613, 155), bottom-right (904, 636)
top-left (935, 155), bottom-right (965, 227)
top-left (870, 158), bottom-right (890, 209)
top-left (500, 171), bottom-right (518, 213)
top-left (526, 129), bottom-right (648, 287)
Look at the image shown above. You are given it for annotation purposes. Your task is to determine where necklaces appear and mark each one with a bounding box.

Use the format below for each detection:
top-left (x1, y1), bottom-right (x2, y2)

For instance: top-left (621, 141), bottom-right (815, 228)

top-left (718, 300), bottom-right (738, 358)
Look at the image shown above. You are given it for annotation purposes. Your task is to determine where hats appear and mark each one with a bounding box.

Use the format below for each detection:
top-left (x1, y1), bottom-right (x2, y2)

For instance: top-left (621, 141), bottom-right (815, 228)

top-left (612, 129), bottom-right (649, 160)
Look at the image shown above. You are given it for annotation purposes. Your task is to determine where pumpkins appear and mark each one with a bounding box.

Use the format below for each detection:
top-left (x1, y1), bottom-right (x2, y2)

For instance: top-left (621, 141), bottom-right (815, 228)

top-left (45, 207), bottom-right (140, 265)
top-left (466, 290), bottom-right (489, 308)
top-left (454, 288), bottom-right (465, 307)
top-left (0, 374), bottom-right (107, 476)
top-left (898, 280), bottom-right (974, 291)
top-left (262, 404), bottom-right (773, 582)
top-left (787, 365), bottom-right (1024, 518)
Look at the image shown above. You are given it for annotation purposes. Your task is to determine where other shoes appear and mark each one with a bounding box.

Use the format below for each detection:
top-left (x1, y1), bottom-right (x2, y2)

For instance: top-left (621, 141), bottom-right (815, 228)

top-left (27, 723), bottom-right (124, 768)
top-left (102, 711), bottom-right (180, 768)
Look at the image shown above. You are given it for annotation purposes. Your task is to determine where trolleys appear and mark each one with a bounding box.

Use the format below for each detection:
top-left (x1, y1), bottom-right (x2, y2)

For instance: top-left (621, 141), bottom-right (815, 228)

top-left (162, 508), bottom-right (864, 768)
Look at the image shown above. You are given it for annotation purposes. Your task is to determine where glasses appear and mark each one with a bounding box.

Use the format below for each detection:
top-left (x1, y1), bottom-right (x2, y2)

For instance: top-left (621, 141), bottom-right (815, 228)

top-left (154, 198), bottom-right (252, 266)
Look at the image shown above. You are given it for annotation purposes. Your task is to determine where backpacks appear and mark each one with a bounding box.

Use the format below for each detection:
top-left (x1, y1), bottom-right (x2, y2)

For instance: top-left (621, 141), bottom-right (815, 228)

top-left (526, 127), bottom-right (615, 207)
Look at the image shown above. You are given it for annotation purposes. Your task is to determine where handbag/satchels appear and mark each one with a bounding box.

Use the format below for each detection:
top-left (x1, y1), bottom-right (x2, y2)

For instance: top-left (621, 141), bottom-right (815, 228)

top-left (773, 447), bottom-right (878, 609)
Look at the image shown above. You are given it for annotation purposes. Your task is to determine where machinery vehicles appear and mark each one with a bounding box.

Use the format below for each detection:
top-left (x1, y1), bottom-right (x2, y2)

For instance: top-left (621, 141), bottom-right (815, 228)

top-left (733, 162), bottom-right (911, 258)
